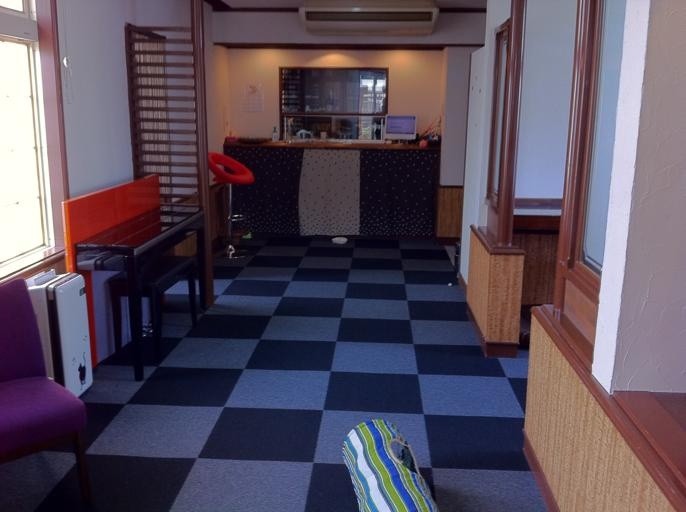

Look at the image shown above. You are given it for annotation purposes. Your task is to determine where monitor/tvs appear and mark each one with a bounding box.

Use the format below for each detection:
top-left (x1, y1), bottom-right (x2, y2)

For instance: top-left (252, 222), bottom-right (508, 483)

top-left (385, 114), bottom-right (417, 144)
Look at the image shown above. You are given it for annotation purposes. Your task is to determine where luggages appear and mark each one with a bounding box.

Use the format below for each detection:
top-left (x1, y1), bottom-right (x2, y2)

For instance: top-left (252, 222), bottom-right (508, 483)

top-left (27, 268), bottom-right (93, 397)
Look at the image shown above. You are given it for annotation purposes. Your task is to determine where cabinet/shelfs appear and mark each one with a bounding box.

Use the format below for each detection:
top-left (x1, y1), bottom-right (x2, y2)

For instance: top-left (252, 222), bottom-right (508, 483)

top-left (224, 138), bottom-right (439, 243)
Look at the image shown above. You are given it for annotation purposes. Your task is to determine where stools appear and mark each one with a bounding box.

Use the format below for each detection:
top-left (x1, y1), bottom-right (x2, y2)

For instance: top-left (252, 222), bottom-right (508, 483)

top-left (108, 252), bottom-right (199, 367)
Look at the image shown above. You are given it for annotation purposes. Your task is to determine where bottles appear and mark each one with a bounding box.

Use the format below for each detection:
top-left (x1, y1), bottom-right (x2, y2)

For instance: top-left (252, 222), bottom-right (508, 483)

top-left (273, 127), bottom-right (278, 142)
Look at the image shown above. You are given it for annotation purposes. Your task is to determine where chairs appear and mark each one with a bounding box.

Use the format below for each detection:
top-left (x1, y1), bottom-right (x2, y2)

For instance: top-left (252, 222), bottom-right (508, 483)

top-left (208, 151), bottom-right (254, 258)
top-left (0, 277), bottom-right (94, 512)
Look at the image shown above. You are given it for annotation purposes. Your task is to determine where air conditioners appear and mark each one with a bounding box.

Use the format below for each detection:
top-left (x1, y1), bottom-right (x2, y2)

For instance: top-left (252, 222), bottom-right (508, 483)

top-left (298, 3), bottom-right (440, 37)
